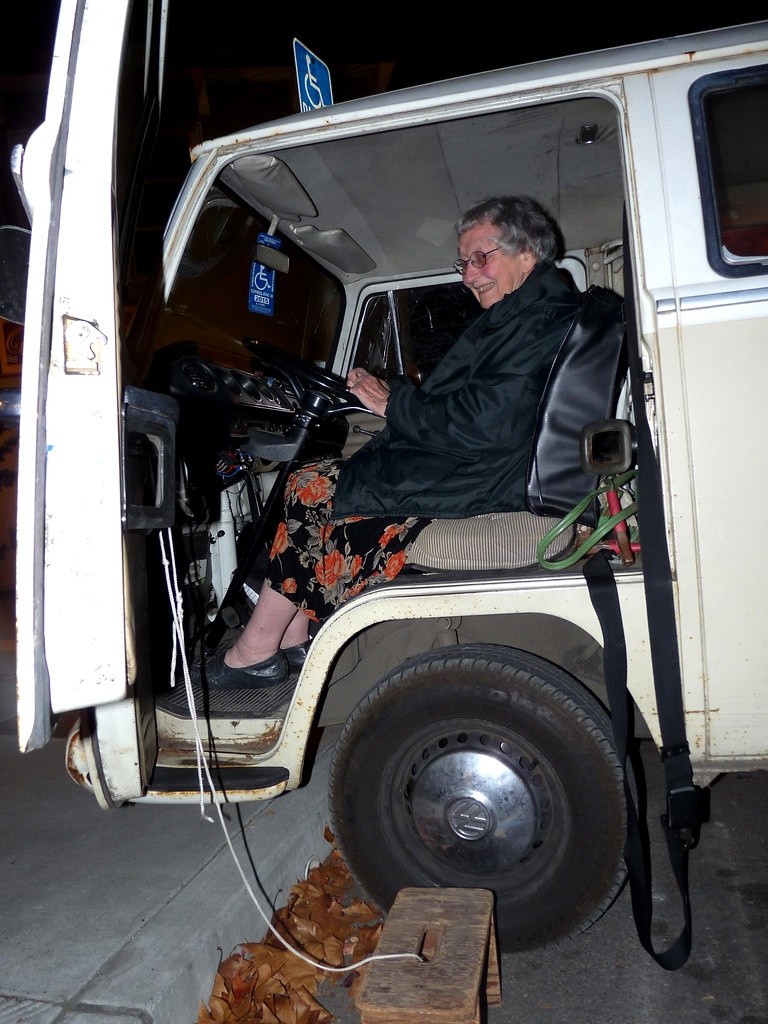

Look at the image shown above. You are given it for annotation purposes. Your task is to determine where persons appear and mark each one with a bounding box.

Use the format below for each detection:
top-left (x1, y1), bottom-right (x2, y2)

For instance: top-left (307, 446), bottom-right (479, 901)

top-left (186, 197), bottom-right (581, 692)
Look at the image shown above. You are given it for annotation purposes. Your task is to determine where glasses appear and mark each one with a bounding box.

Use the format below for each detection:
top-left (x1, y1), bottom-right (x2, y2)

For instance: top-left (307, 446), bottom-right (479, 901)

top-left (453, 248), bottom-right (499, 275)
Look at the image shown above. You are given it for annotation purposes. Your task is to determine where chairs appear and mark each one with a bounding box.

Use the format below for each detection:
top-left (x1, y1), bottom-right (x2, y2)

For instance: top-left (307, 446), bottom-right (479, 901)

top-left (361, 284), bottom-right (630, 571)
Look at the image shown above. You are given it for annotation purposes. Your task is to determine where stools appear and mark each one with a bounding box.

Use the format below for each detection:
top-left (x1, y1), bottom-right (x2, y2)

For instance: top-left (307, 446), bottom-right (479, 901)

top-left (356, 887), bottom-right (504, 1024)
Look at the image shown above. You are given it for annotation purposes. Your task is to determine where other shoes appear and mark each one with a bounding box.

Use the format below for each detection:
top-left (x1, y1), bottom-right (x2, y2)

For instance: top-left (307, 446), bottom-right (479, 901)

top-left (188, 652), bottom-right (289, 690)
top-left (282, 640), bottom-right (311, 670)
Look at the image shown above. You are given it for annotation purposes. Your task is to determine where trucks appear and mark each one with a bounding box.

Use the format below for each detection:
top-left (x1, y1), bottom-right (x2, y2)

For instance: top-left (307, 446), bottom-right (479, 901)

top-left (8, 0), bottom-right (766, 960)
